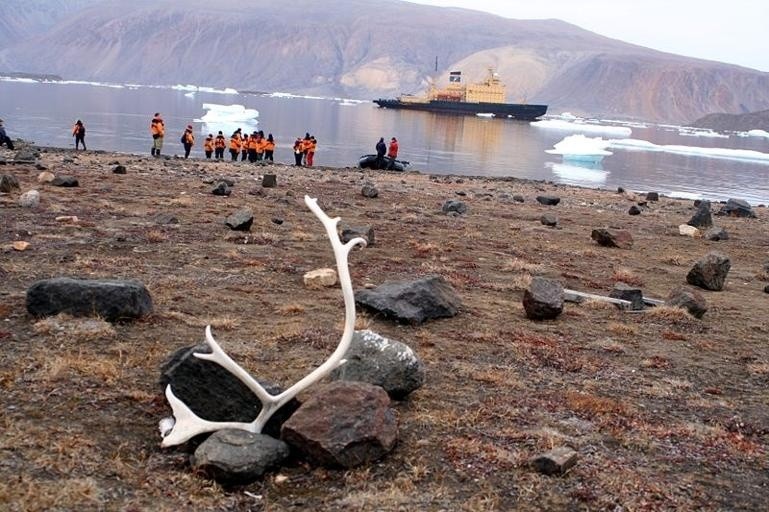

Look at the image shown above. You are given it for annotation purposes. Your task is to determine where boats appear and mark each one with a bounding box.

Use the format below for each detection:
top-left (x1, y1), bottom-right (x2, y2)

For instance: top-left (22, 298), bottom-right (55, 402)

top-left (357, 153), bottom-right (410, 172)
top-left (370, 62), bottom-right (551, 121)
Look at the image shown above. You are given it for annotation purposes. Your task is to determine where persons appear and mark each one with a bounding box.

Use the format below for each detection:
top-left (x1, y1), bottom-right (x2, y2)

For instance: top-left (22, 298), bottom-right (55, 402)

top-left (376, 137), bottom-right (387, 169)
top-left (73, 120), bottom-right (86, 151)
top-left (0, 119), bottom-right (14, 150)
top-left (386, 137), bottom-right (398, 170)
top-left (294, 133), bottom-right (317, 168)
top-left (151, 113), bottom-right (164, 156)
top-left (184, 125), bottom-right (194, 158)
top-left (205, 128), bottom-right (275, 163)
